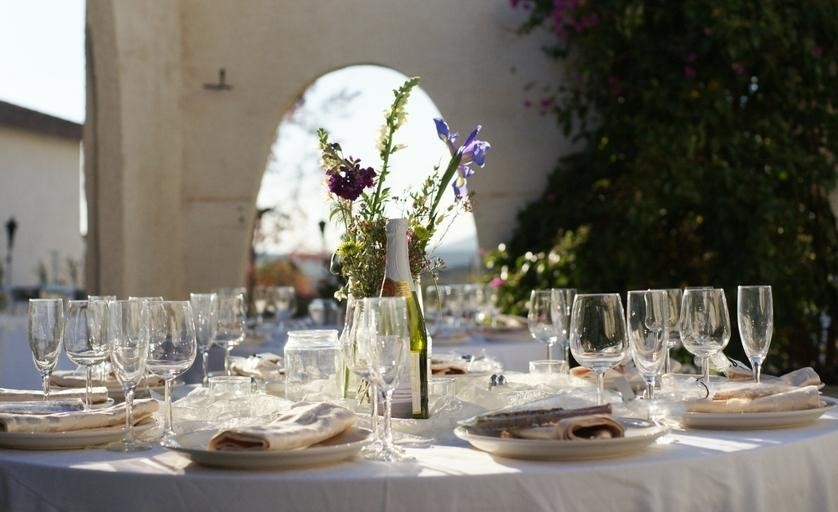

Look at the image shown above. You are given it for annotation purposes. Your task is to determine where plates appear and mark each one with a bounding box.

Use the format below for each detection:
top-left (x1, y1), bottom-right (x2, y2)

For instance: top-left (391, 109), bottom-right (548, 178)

top-left (0, 418), bottom-right (136, 456)
top-left (157, 424), bottom-right (379, 469)
top-left (451, 415), bottom-right (672, 461)
top-left (674, 395), bottom-right (838, 429)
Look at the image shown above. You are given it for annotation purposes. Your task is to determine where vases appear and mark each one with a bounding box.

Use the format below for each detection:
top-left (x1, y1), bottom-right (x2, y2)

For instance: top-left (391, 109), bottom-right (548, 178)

top-left (339, 293), bottom-right (431, 420)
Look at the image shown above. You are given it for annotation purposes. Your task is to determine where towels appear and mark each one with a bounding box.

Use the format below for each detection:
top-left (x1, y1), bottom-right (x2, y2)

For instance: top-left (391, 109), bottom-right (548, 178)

top-left (209, 401), bottom-right (359, 453)
top-left (684, 387), bottom-right (821, 416)
top-left (50, 371), bottom-right (167, 391)
top-left (227, 352), bottom-right (281, 383)
top-left (0, 400), bottom-right (158, 432)
top-left (573, 364), bottom-right (645, 388)
top-left (502, 404), bottom-right (625, 441)
top-left (0, 388), bottom-right (105, 409)
top-left (712, 368), bottom-right (821, 399)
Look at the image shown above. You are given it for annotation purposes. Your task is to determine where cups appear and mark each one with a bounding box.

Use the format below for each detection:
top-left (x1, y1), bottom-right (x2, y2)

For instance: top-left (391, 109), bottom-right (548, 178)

top-left (624, 288), bottom-right (683, 414)
top-left (529, 359), bottom-right (569, 384)
top-left (146, 301), bottom-right (197, 441)
top-left (27, 298), bottom-right (66, 404)
top-left (551, 288), bottom-right (578, 376)
top-left (527, 288), bottom-right (566, 373)
top-left (105, 296), bottom-right (167, 452)
top-left (425, 283), bottom-right (500, 328)
top-left (206, 375), bottom-right (252, 421)
top-left (61, 293), bottom-right (116, 416)
top-left (283, 330), bottom-right (345, 409)
top-left (679, 286), bottom-right (732, 392)
top-left (339, 295), bottom-right (416, 462)
top-left (211, 291), bottom-right (248, 376)
top-left (737, 284), bottom-right (774, 386)
top-left (189, 290), bottom-right (220, 388)
top-left (252, 285), bottom-right (295, 326)
top-left (569, 293), bottom-right (629, 415)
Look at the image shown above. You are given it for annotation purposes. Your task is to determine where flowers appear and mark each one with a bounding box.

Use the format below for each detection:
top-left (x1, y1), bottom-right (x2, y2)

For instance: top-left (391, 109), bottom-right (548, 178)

top-left (315, 77), bottom-right (490, 293)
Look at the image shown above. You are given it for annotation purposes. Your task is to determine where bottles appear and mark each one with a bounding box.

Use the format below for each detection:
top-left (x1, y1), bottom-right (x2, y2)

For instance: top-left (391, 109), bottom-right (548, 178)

top-left (377, 219), bottom-right (427, 420)
